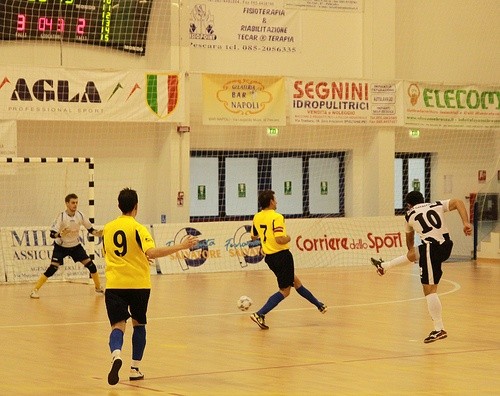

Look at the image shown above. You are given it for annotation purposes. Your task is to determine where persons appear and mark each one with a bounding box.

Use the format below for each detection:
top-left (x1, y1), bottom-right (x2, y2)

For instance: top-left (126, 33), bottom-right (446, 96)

top-left (29, 193), bottom-right (103, 300)
top-left (370, 190), bottom-right (473, 343)
top-left (248, 190), bottom-right (328, 330)
top-left (100, 189), bottom-right (199, 385)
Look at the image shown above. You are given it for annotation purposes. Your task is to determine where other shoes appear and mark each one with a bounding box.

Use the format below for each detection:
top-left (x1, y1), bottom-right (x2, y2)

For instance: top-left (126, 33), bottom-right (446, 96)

top-left (129, 369), bottom-right (144, 380)
top-left (29, 290), bottom-right (40, 299)
top-left (96, 290), bottom-right (104, 294)
top-left (108, 356), bottom-right (122, 385)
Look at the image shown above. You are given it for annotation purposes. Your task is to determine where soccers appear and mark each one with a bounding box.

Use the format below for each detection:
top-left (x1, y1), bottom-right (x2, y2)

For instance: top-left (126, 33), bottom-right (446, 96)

top-left (237, 296), bottom-right (252, 311)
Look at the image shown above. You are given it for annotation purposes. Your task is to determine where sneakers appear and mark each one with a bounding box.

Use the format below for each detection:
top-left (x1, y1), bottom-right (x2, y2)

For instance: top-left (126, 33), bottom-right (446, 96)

top-left (424, 330), bottom-right (447, 343)
top-left (318, 304), bottom-right (327, 314)
top-left (371, 258), bottom-right (384, 276)
top-left (250, 313), bottom-right (269, 330)
